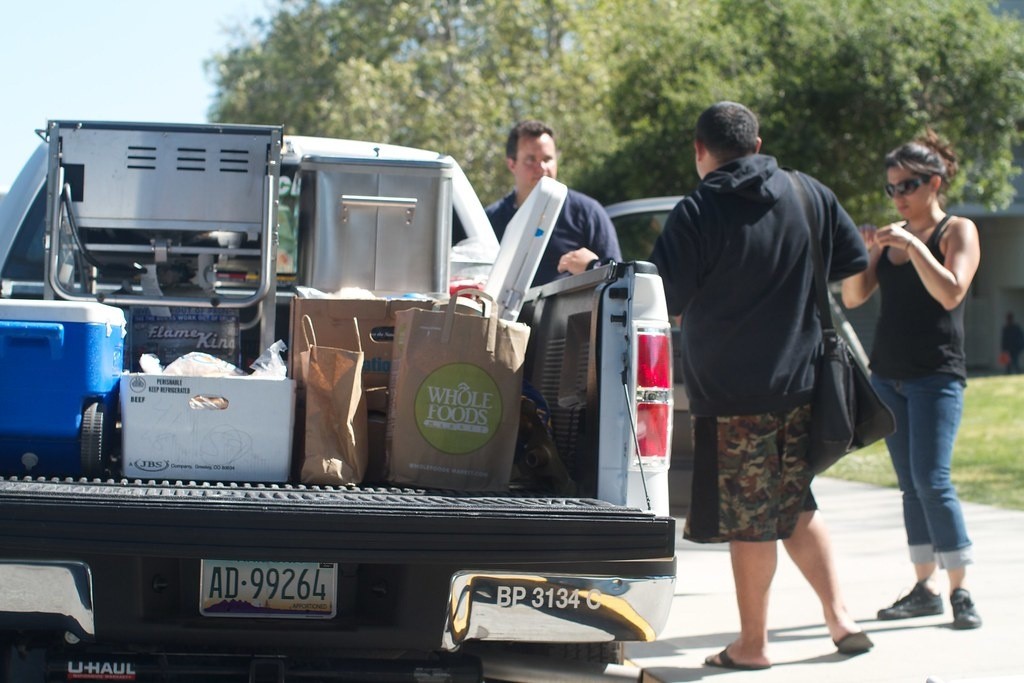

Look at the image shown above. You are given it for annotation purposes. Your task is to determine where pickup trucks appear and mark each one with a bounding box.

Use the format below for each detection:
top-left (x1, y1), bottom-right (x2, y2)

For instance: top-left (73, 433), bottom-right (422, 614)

top-left (0, 119), bottom-right (689, 683)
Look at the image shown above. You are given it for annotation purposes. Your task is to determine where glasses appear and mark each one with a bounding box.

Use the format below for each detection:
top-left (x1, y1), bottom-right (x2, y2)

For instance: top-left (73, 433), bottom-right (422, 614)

top-left (885, 173), bottom-right (927, 197)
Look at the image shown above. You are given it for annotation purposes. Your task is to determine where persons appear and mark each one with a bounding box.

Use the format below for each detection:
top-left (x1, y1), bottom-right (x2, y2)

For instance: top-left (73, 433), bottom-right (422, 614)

top-left (840, 124), bottom-right (983, 630)
top-left (482, 121), bottom-right (624, 288)
top-left (557, 100), bottom-right (874, 671)
top-left (1001, 313), bottom-right (1024, 375)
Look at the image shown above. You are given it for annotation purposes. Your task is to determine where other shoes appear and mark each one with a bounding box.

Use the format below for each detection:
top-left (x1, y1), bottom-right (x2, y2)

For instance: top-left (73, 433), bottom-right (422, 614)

top-left (949, 588), bottom-right (982, 629)
top-left (877, 579), bottom-right (944, 621)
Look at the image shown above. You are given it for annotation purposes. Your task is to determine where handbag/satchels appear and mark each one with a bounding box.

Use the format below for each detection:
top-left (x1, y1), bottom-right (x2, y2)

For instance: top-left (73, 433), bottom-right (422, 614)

top-left (299, 314), bottom-right (369, 486)
top-left (385, 288), bottom-right (531, 492)
top-left (809, 330), bottom-right (896, 473)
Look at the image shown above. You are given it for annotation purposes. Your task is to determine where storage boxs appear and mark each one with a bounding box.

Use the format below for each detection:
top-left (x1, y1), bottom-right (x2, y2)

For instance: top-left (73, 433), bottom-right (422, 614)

top-left (0, 300), bottom-right (297, 483)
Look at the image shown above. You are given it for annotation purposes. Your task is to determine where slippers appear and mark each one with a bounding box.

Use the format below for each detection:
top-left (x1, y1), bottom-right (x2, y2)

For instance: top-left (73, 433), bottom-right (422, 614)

top-left (832, 629), bottom-right (875, 654)
top-left (705, 642), bottom-right (772, 670)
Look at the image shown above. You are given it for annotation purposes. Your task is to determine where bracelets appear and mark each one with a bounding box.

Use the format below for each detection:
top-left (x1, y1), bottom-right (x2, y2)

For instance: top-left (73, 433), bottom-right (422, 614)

top-left (906, 236), bottom-right (916, 252)
